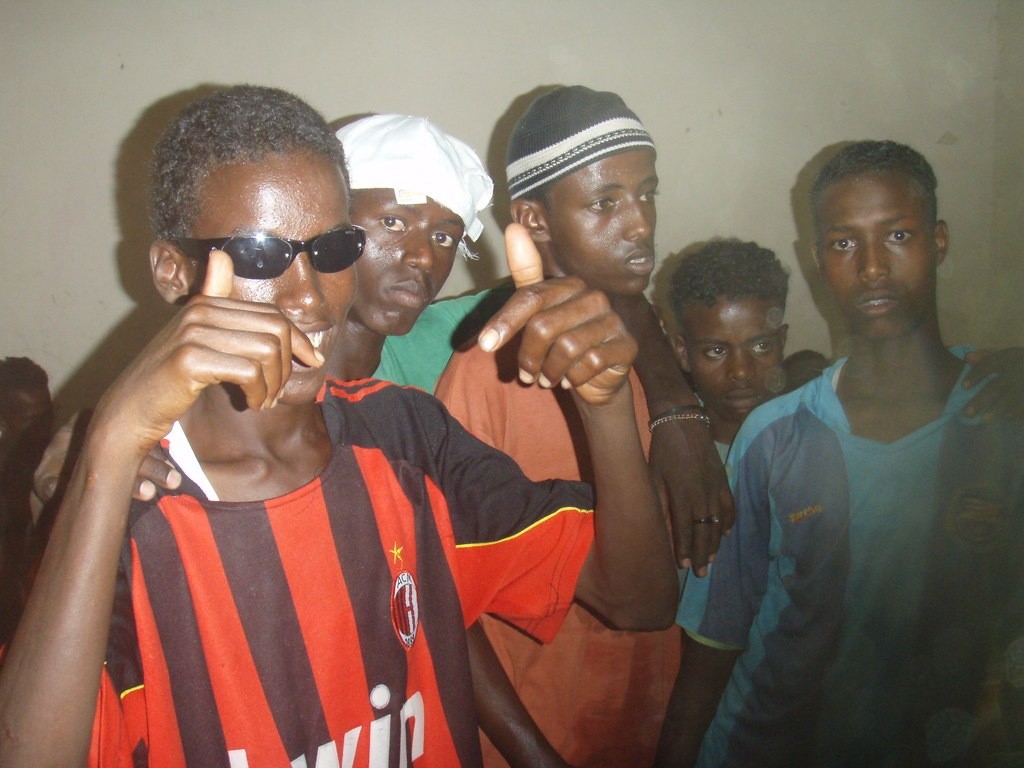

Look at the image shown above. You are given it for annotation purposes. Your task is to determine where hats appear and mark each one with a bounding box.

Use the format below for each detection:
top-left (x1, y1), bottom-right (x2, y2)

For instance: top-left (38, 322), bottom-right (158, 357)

top-left (335, 114), bottom-right (494, 242)
top-left (506, 84), bottom-right (658, 201)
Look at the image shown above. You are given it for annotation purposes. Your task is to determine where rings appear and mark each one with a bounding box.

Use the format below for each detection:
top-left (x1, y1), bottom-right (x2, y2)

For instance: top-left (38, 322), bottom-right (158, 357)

top-left (695, 514), bottom-right (719, 524)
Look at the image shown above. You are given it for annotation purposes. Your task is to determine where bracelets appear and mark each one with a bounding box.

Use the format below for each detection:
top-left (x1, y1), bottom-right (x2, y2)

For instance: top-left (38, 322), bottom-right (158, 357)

top-left (649, 404), bottom-right (710, 430)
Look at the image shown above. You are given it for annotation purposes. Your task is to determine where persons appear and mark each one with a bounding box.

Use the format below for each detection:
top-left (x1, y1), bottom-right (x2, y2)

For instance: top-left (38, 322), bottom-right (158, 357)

top-left (24, 113), bottom-right (734, 579)
top-left (656, 140), bottom-right (1024, 768)
top-left (0, 357), bottom-right (54, 645)
top-left (433, 87), bottom-right (682, 768)
top-left (0, 82), bottom-right (677, 768)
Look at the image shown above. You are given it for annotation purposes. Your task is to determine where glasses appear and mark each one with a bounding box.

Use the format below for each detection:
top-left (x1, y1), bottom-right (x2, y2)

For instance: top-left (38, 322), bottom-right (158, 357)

top-left (172, 224), bottom-right (366, 280)
